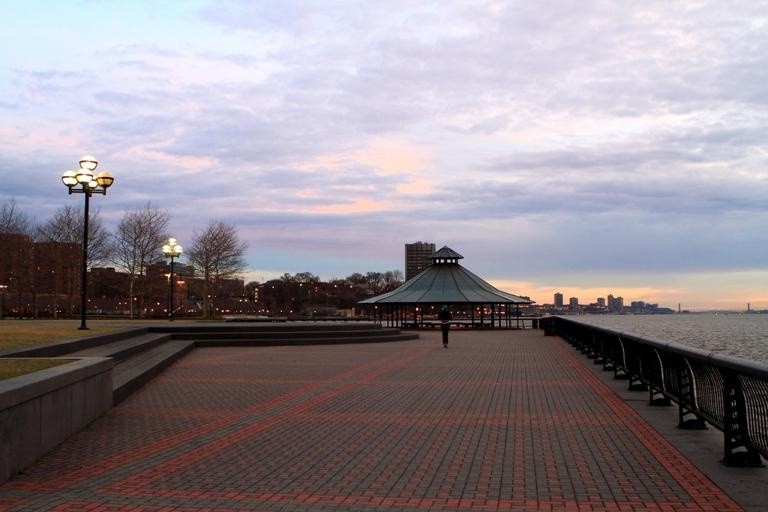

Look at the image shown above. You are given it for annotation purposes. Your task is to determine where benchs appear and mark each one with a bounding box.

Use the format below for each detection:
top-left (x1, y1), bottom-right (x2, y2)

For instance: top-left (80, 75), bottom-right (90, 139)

top-left (401, 321), bottom-right (490, 328)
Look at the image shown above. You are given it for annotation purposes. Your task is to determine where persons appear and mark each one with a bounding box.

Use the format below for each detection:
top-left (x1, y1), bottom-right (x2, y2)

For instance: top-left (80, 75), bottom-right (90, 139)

top-left (440, 304), bottom-right (451, 350)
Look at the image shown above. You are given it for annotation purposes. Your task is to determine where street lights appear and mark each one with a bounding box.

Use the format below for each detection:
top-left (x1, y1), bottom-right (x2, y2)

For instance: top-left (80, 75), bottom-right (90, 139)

top-left (176, 280), bottom-right (184, 314)
top-left (161, 237), bottom-right (183, 321)
top-left (0, 284), bottom-right (8, 320)
top-left (60, 152), bottom-right (115, 331)
top-left (162, 273), bottom-right (177, 314)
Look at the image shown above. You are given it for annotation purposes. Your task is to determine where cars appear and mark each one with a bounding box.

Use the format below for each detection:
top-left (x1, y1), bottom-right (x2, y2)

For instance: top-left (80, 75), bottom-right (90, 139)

top-left (86, 305), bottom-right (103, 314)
top-left (112, 307), bottom-right (131, 315)
